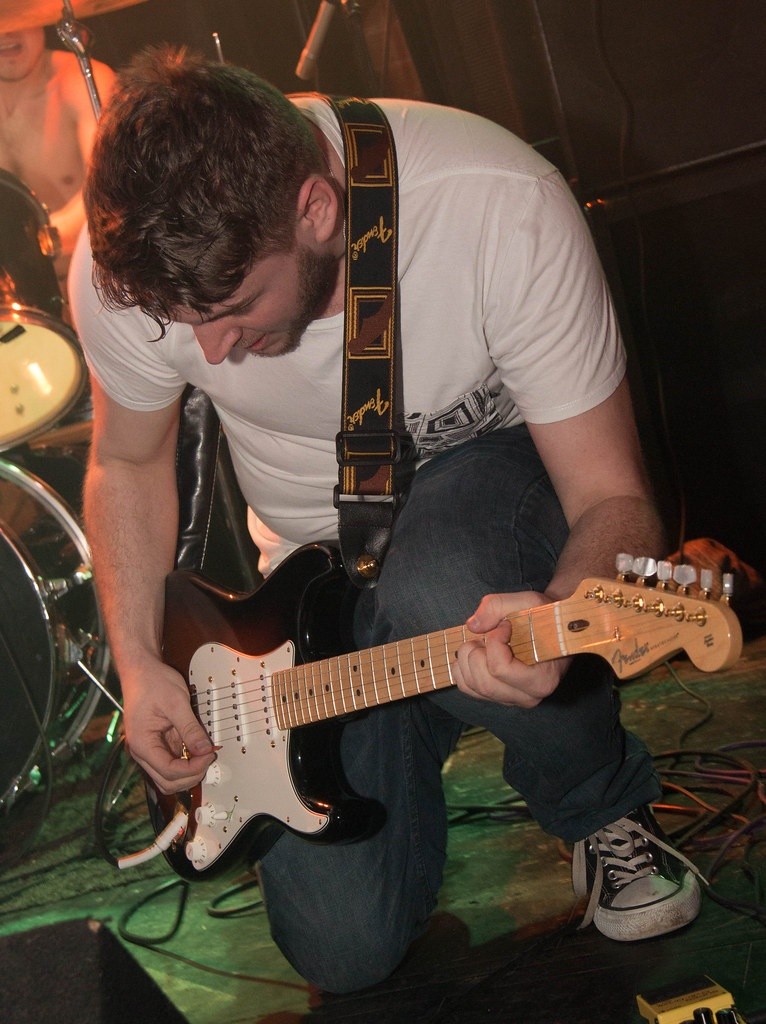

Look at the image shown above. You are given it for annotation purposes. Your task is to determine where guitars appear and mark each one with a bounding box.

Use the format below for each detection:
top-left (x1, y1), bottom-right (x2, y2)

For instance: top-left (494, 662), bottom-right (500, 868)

top-left (144, 538), bottom-right (747, 885)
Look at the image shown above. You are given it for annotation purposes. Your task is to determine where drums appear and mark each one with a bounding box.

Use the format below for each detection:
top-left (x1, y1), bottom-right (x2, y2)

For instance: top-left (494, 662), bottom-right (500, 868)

top-left (0, 164), bottom-right (89, 452)
top-left (0, 454), bottom-right (113, 816)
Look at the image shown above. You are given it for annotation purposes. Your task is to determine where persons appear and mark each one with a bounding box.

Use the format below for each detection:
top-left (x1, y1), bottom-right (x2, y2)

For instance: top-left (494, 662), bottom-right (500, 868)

top-left (0, 26), bottom-right (123, 423)
top-left (67, 48), bottom-right (709, 996)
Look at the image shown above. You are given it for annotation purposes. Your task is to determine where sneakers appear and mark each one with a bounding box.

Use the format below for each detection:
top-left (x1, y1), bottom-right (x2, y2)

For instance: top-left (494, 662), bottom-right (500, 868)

top-left (569, 800), bottom-right (713, 945)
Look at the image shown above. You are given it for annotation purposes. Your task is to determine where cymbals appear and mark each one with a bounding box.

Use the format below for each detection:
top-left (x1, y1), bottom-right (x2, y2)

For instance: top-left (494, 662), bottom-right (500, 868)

top-left (0, 0), bottom-right (143, 35)
